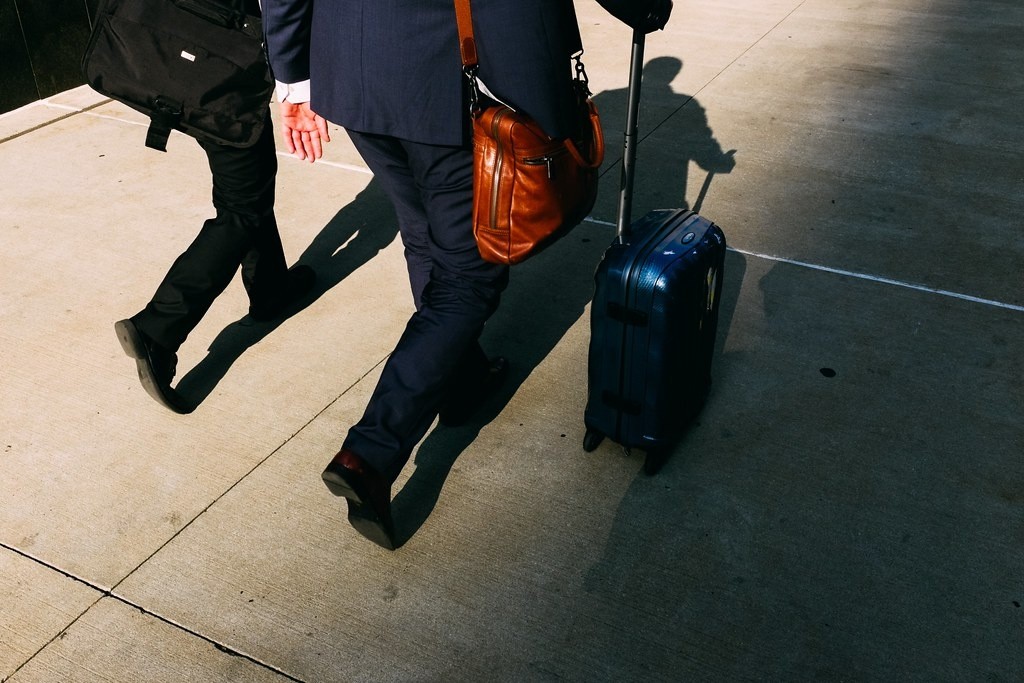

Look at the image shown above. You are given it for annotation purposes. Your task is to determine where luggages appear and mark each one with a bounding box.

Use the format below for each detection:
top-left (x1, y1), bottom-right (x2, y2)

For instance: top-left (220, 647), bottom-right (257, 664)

top-left (583, 27), bottom-right (726, 475)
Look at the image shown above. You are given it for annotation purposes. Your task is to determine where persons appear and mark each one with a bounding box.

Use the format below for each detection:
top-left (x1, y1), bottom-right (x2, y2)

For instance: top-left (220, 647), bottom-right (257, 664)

top-left (113, 0), bottom-right (315, 414)
top-left (260, 0), bottom-right (675, 548)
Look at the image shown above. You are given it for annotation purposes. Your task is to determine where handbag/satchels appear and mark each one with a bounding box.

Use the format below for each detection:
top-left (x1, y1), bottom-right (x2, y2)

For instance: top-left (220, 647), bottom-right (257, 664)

top-left (80, 0), bottom-right (276, 147)
top-left (470, 97), bottom-right (605, 263)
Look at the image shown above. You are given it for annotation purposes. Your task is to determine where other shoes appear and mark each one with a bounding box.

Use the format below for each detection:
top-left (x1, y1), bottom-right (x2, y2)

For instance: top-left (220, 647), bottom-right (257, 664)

top-left (115, 316), bottom-right (184, 413)
top-left (441, 356), bottom-right (512, 423)
top-left (249, 262), bottom-right (317, 319)
top-left (322, 451), bottom-right (397, 550)
